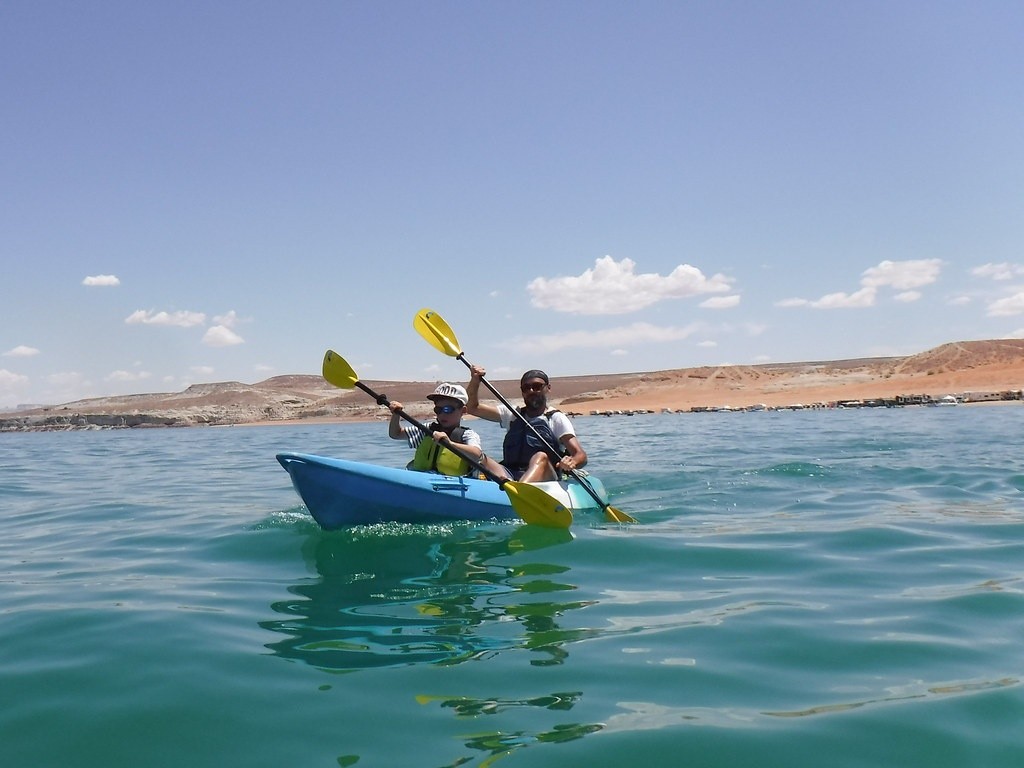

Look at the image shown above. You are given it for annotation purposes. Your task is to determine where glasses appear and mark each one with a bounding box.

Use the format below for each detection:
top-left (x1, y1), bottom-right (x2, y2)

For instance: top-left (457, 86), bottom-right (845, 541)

top-left (433, 406), bottom-right (456, 414)
top-left (520, 382), bottom-right (548, 392)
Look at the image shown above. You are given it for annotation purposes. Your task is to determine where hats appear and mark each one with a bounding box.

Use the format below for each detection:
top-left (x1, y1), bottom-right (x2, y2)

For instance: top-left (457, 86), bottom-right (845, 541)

top-left (520, 369), bottom-right (549, 384)
top-left (426, 383), bottom-right (469, 405)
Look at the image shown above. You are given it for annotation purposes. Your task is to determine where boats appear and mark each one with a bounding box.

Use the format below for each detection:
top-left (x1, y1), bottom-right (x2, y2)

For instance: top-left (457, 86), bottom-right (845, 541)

top-left (275, 451), bottom-right (609, 529)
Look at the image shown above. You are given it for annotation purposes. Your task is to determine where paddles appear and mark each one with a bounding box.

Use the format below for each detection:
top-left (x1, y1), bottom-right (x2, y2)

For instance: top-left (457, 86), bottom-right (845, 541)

top-left (322, 349), bottom-right (573, 528)
top-left (413, 308), bottom-right (636, 524)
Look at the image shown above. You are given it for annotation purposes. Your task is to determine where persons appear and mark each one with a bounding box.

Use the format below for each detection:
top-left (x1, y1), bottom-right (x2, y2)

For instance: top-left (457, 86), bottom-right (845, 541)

top-left (466, 365), bottom-right (589, 484)
top-left (389, 382), bottom-right (482, 479)
